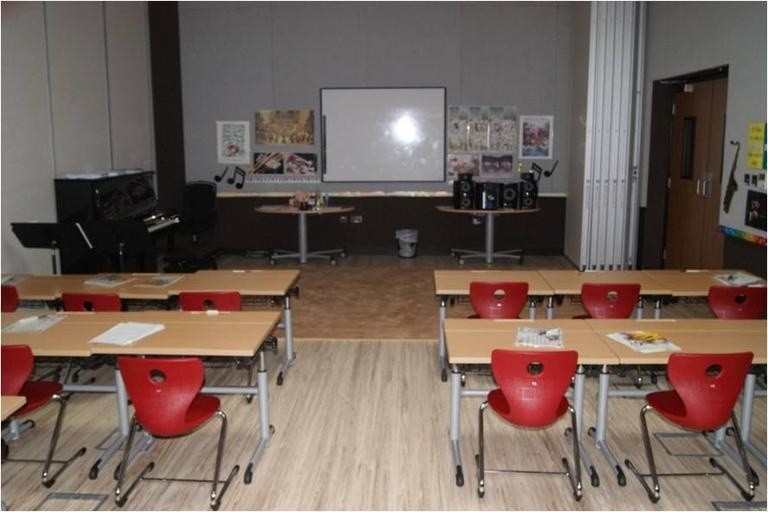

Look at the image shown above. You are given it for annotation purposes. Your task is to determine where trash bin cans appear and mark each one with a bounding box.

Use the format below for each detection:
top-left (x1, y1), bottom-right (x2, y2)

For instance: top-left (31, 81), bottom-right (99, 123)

top-left (396, 229), bottom-right (419, 259)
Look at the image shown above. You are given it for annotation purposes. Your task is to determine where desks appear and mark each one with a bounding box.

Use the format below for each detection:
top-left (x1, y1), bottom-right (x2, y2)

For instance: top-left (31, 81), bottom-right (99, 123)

top-left (4, 268), bottom-right (302, 386)
top-left (440, 319), bottom-right (767, 486)
top-left (435, 267), bottom-right (768, 384)
top-left (438, 206), bottom-right (533, 265)
top-left (256, 206), bottom-right (359, 265)
top-left (3, 311), bottom-right (286, 488)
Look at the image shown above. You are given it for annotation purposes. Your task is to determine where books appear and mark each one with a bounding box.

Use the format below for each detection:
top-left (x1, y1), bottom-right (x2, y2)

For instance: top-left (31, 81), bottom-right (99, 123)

top-left (1, 314), bottom-right (67, 333)
top-left (83, 271), bottom-right (137, 289)
top-left (132, 274), bottom-right (184, 289)
top-left (713, 270), bottom-right (762, 288)
top-left (1, 274), bottom-right (28, 286)
top-left (606, 330), bottom-right (681, 354)
top-left (88, 321), bottom-right (165, 346)
top-left (514, 325), bottom-right (564, 349)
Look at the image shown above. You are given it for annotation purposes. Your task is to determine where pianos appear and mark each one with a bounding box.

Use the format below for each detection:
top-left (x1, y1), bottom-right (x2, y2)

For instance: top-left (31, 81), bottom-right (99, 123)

top-left (54, 169), bottom-right (182, 273)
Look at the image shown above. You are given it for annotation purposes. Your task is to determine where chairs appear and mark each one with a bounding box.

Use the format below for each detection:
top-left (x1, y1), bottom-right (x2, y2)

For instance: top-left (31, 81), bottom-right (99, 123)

top-left (114, 355), bottom-right (244, 512)
top-left (711, 285), bottom-right (767, 387)
top-left (441, 279), bottom-right (530, 387)
top-left (177, 290), bottom-right (279, 404)
top-left (165, 181), bottom-right (220, 268)
top-left (60, 291), bottom-right (125, 369)
top-left (1, 344), bottom-right (88, 490)
top-left (471, 348), bottom-right (587, 500)
top-left (1, 285), bottom-right (22, 315)
top-left (623, 350), bottom-right (760, 499)
top-left (577, 282), bottom-right (659, 386)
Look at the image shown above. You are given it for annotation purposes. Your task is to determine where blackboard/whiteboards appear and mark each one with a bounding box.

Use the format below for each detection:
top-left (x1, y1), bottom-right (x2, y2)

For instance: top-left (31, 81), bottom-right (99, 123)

top-left (319, 86), bottom-right (447, 182)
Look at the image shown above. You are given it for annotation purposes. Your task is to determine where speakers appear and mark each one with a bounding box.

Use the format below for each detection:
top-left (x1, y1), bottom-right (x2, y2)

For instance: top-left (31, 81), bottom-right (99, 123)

top-left (453, 172), bottom-right (537, 210)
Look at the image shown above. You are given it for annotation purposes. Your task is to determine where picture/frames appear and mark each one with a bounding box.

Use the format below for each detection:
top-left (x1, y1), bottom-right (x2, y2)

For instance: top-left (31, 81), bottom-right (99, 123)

top-left (519, 114), bottom-right (555, 161)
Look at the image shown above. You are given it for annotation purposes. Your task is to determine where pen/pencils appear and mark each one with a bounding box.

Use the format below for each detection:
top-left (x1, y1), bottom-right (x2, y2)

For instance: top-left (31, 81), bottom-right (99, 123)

top-left (18, 315), bottom-right (47, 323)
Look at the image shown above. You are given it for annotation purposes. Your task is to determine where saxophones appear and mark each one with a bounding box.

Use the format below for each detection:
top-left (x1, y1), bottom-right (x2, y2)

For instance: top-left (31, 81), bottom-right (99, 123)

top-left (722, 141), bottom-right (741, 214)
